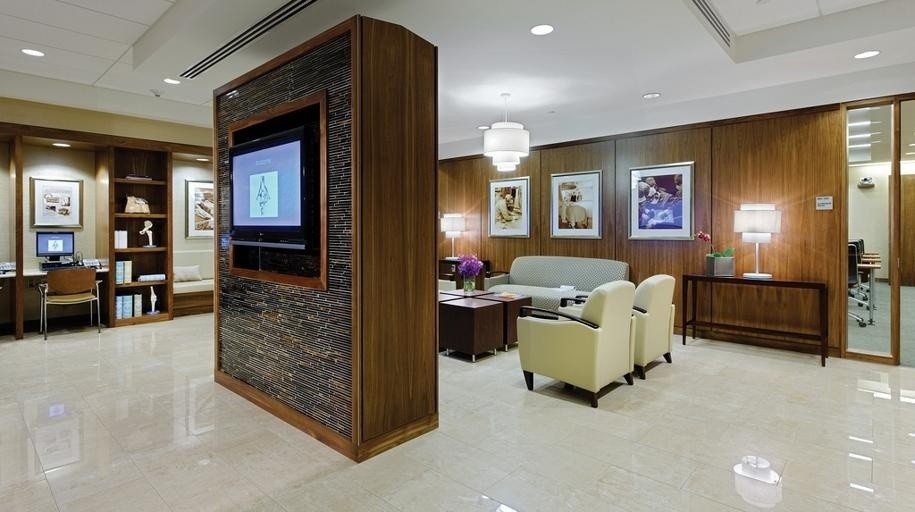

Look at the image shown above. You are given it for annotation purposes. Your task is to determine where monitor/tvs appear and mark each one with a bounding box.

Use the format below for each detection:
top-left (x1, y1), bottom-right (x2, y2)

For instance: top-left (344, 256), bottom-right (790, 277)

top-left (227, 126), bottom-right (311, 253)
top-left (35, 230), bottom-right (74, 262)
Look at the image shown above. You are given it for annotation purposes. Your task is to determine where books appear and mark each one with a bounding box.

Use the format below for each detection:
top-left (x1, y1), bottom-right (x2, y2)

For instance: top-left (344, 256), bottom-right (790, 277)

top-left (114, 229), bottom-right (143, 321)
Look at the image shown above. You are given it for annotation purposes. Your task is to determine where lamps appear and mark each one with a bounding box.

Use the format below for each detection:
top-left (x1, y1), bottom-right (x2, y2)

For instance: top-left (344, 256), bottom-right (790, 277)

top-left (482, 92), bottom-right (529, 172)
top-left (732, 453), bottom-right (782, 511)
top-left (733, 202), bottom-right (781, 279)
top-left (439, 213), bottom-right (465, 260)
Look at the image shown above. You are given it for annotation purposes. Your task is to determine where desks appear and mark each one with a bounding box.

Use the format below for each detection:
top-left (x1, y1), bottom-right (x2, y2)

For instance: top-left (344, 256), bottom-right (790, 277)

top-left (22, 258), bottom-right (110, 280)
top-left (865, 252), bottom-right (879, 255)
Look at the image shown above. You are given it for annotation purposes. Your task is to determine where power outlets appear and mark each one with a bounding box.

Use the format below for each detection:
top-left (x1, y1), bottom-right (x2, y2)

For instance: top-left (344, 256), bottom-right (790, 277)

top-left (816, 196), bottom-right (833, 210)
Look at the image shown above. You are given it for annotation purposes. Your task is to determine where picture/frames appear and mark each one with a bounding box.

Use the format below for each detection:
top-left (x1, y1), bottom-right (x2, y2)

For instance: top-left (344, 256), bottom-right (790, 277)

top-left (487, 176), bottom-right (530, 239)
top-left (549, 169), bottom-right (604, 241)
top-left (628, 161), bottom-right (696, 241)
top-left (29, 176), bottom-right (84, 229)
top-left (184, 179), bottom-right (216, 241)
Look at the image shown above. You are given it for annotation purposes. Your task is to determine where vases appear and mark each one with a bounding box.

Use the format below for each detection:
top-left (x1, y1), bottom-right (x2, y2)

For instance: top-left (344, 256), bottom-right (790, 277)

top-left (461, 274), bottom-right (476, 294)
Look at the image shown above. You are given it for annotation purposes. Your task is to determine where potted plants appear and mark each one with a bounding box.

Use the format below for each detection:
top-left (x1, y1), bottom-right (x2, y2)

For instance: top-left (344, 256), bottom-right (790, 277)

top-left (705, 254), bottom-right (736, 278)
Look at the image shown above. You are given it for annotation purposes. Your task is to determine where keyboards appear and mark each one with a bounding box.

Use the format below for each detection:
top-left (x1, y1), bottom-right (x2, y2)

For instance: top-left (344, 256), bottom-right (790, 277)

top-left (41, 261), bottom-right (86, 272)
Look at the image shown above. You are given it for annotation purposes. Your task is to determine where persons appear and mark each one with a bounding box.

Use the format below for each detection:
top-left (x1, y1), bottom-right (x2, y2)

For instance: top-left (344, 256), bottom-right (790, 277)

top-left (495, 194), bottom-right (520, 224)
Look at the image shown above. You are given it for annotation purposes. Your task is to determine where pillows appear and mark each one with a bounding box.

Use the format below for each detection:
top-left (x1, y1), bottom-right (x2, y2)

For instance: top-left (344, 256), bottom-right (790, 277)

top-left (173, 264), bottom-right (202, 283)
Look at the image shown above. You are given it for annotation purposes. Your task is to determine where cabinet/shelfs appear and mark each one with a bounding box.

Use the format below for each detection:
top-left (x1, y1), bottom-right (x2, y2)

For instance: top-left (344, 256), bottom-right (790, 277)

top-left (889, 174), bottom-right (915, 286)
top-left (95, 147), bottom-right (173, 327)
top-left (682, 273), bottom-right (829, 367)
top-left (439, 260), bottom-right (489, 290)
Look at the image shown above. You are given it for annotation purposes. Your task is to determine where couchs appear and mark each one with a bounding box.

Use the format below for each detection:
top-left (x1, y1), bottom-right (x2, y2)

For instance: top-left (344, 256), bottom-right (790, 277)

top-left (173, 247), bottom-right (215, 316)
top-left (484, 255), bottom-right (629, 321)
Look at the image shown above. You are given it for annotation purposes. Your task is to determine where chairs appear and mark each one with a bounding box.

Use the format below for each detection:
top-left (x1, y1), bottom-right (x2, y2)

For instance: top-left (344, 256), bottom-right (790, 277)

top-left (39, 267), bottom-right (102, 340)
top-left (575, 273), bottom-right (675, 380)
top-left (848, 240), bottom-right (881, 326)
top-left (848, 237), bottom-right (876, 328)
top-left (437, 273), bottom-right (456, 291)
top-left (516, 280), bottom-right (635, 408)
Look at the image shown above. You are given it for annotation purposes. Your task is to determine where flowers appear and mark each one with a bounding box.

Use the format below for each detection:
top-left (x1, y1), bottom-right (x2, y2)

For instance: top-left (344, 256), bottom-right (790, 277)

top-left (697, 231), bottom-right (734, 257)
top-left (458, 252), bottom-right (483, 276)
